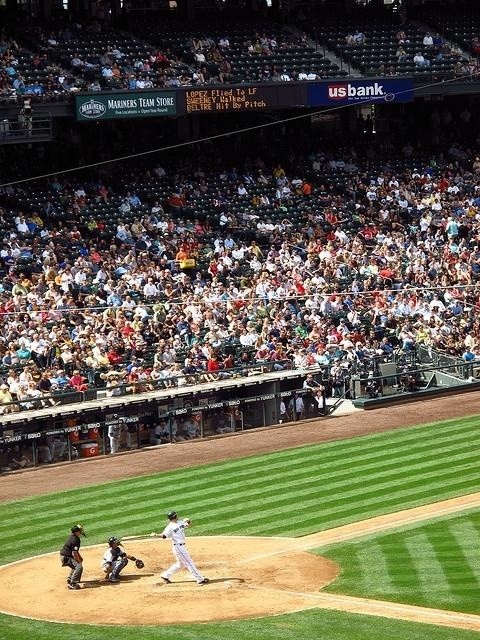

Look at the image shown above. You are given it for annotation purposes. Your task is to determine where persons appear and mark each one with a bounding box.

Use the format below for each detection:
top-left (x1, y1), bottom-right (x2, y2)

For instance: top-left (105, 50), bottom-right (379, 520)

top-left (60, 525), bottom-right (87, 589)
top-left (0, 146), bottom-right (480, 473)
top-left (101, 537), bottom-right (142, 583)
top-left (0, 30), bottom-right (480, 102)
top-left (150, 511), bottom-right (208, 584)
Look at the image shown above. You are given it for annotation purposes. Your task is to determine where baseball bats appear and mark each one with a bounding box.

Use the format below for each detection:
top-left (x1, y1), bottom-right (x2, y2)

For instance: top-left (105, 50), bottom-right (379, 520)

top-left (121, 533), bottom-right (157, 541)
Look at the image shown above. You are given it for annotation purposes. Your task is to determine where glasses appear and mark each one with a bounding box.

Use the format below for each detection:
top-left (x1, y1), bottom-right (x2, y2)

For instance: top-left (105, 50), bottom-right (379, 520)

top-left (1, 174), bottom-right (479, 390)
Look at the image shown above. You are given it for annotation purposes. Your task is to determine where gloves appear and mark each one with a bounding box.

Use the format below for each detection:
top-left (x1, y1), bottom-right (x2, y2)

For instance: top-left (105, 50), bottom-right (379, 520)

top-left (150, 533), bottom-right (158, 537)
top-left (183, 518), bottom-right (191, 523)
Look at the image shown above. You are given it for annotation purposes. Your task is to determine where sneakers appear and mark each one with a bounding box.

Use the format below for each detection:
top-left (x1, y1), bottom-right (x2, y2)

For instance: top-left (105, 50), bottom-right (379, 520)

top-left (161, 576), bottom-right (172, 583)
top-left (109, 576), bottom-right (121, 583)
top-left (198, 578), bottom-right (209, 584)
top-left (66, 578), bottom-right (80, 589)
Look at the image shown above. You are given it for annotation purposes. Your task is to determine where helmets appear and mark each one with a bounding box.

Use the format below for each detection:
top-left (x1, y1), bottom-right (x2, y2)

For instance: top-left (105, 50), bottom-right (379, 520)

top-left (108, 536), bottom-right (119, 545)
top-left (71, 525), bottom-right (83, 533)
top-left (168, 512), bottom-right (177, 519)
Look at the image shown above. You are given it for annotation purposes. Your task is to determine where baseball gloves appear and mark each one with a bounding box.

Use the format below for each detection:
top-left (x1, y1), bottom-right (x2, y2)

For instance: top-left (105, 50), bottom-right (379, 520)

top-left (135, 559), bottom-right (144, 569)
